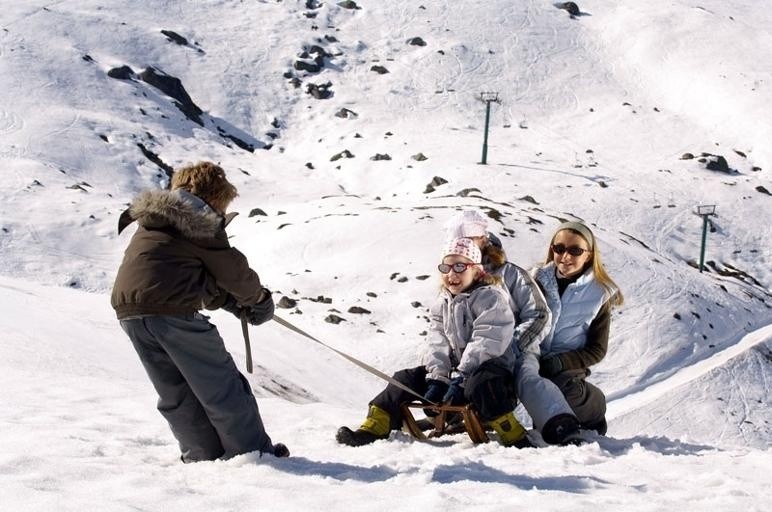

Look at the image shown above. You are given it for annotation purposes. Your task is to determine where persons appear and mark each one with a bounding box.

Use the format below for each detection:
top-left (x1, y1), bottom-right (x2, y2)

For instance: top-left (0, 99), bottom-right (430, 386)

top-left (335, 238), bottom-right (534, 451)
top-left (110, 161), bottom-right (291, 463)
top-left (450, 207), bottom-right (589, 449)
top-left (533, 222), bottom-right (625, 436)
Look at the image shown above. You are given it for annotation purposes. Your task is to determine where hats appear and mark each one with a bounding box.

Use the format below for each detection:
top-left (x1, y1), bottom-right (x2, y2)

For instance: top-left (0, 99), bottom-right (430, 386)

top-left (442, 238), bottom-right (483, 273)
top-left (447, 210), bottom-right (488, 245)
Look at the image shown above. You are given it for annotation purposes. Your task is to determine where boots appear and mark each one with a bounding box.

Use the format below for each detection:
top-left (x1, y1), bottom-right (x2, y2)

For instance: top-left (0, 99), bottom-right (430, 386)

top-left (541, 413), bottom-right (586, 446)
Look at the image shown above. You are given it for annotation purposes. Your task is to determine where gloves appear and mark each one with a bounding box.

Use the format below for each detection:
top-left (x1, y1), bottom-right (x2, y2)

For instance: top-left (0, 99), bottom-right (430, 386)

top-left (221, 287), bottom-right (274, 324)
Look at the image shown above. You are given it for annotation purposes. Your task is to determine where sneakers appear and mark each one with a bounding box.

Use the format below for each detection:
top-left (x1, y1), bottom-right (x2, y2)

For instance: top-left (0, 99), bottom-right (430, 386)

top-left (336, 426), bottom-right (390, 447)
top-left (505, 436), bottom-right (537, 448)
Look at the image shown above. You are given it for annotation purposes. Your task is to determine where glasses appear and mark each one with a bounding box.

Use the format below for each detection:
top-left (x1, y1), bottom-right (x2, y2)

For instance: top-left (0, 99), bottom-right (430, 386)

top-left (438, 262), bottom-right (482, 274)
top-left (551, 244), bottom-right (592, 256)
